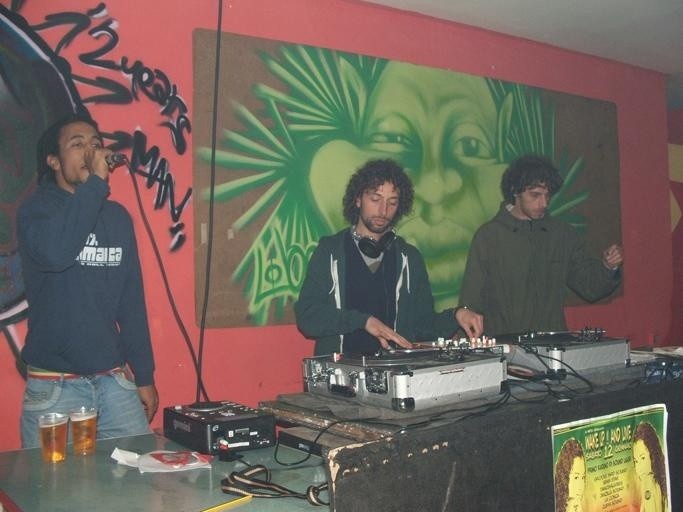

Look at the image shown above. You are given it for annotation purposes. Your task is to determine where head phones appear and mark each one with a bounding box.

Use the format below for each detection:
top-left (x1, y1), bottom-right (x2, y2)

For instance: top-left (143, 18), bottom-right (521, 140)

top-left (351, 206), bottom-right (394, 258)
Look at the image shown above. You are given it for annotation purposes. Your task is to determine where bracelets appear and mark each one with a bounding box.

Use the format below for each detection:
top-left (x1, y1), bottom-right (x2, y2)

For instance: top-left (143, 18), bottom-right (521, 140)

top-left (454, 304), bottom-right (470, 318)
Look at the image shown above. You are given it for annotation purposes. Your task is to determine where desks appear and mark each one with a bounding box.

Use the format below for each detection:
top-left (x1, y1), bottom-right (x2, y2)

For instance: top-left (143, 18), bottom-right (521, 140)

top-left (259, 377), bottom-right (683, 512)
top-left (0, 432), bottom-right (331, 512)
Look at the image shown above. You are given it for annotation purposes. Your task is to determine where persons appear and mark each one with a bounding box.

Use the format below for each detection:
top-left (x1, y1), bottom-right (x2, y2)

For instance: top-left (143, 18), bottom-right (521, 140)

top-left (632, 420), bottom-right (668, 511)
top-left (18, 113), bottom-right (159, 447)
top-left (552, 436), bottom-right (588, 511)
top-left (458, 152), bottom-right (625, 340)
top-left (296, 159), bottom-right (485, 356)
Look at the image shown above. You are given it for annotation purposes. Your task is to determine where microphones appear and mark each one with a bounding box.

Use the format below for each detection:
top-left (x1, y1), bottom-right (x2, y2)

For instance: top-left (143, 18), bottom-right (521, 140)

top-left (105, 154), bottom-right (126, 164)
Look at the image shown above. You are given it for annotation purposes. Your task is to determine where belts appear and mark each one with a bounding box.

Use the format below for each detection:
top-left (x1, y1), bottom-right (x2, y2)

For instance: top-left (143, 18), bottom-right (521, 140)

top-left (27, 364), bottom-right (126, 378)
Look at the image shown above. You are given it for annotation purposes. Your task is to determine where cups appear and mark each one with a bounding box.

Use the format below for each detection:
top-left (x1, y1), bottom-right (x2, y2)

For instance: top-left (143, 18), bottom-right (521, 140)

top-left (67, 410), bottom-right (97, 455)
top-left (36, 412), bottom-right (68, 462)
